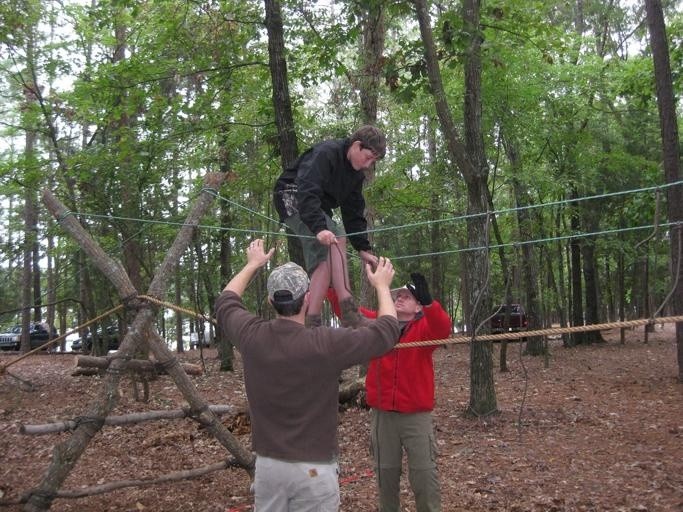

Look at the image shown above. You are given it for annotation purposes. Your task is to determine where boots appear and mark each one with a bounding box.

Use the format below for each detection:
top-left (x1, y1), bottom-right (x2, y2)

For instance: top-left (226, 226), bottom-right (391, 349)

top-left (339, 297), bottom-right (376, 328)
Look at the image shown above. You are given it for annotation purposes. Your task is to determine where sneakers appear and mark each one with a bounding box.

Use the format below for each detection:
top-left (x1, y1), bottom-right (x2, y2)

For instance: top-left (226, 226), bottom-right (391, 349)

top-left (305, 314), bottom-right (321, 329)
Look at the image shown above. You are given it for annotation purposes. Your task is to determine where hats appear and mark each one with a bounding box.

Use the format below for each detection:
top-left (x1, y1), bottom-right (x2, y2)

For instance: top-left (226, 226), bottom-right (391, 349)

top-left (267, 262), bottom-right (310, 305)
top-left (390, 284), bottom-right (416, 300)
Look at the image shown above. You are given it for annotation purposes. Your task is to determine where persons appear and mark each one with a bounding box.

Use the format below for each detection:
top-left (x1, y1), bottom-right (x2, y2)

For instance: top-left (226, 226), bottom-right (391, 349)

top-left (213, 239), bottom-right (398, 512)
top-left (273, 126), bottom-right (385, 329)
top-left (326, 273), bottom-right (453, 512)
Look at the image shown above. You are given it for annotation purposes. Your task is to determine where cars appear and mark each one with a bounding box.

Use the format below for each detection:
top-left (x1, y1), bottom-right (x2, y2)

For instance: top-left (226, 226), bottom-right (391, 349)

top-left (71, 326), bottom-right (120, 351)
top-left (490, 304), bottom-right (529, 342)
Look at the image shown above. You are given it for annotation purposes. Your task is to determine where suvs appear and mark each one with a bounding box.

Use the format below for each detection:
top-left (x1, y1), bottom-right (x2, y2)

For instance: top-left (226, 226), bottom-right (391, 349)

top-left (0, 323), bottom-right (60, 351)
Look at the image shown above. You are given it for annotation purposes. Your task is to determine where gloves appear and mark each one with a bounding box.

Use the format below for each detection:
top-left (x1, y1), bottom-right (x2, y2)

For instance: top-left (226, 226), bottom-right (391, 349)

top-left (406, 272), bottom-right (432, 305)
